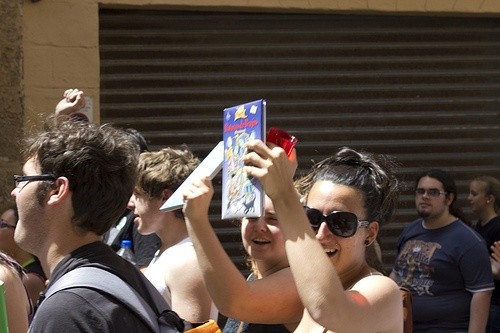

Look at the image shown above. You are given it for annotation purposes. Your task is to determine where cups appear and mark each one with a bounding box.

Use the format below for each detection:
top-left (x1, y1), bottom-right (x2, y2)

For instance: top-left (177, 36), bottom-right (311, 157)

top-left (266, 128), bottom-right (297, 158)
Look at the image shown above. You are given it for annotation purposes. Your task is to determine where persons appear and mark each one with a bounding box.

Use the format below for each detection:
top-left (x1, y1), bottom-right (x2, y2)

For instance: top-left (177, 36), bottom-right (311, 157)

top-left (243, 139), bottom-right (403, 333)
top-left (56, 88), bottom-right (90, 129)
top-left (126, 148), bottom-right (221, 333)
top-left (11, 121), bottom-right (192, 333)
top-left (183, 169), bottom-right (305, 333)
top-left (491, 241), bottom-right (500, 275)
top-left (468, 175), bottom-right (500, 333)
top-left (390, 170), bottom-right (494, 333)
top-left (0, 202), bottom-right (47, 333)
top-left (0, 252), bottom-right (34, 333)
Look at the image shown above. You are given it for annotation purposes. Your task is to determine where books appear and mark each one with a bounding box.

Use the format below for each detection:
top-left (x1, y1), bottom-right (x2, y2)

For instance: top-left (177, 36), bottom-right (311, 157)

top-left (222, 98), bottom-right (266, 219)
top-left (159, 140), bottom-right (224, 212)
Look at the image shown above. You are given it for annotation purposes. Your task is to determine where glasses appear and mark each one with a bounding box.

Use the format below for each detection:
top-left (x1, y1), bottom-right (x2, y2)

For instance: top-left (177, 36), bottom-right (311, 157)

top-left (302, 205), bottom-right (370, 238)
top-left (414, 187), bottom-right (448, 197)
top-left (0, 222), bottom-right (17, 229)
top-left (14, 174), bottom-right (57, 187)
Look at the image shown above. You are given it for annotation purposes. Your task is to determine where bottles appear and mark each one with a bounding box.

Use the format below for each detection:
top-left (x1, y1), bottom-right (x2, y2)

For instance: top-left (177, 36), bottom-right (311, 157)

top-left (117, 240), bottom-right (133, 262)
